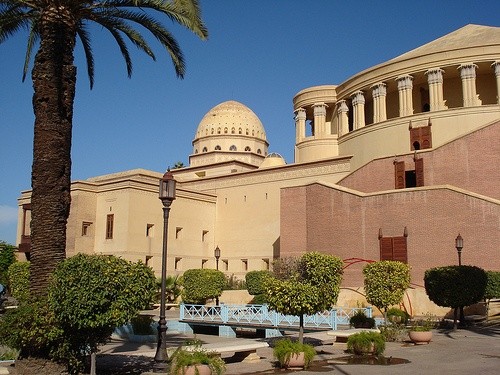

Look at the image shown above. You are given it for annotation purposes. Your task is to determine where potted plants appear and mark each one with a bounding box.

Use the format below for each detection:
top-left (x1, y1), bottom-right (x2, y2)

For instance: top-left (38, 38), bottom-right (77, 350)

top-left (274, 337), bottom-right (316, 371)
top-left (168, 346), bottom-right (226, 375)
top-left (408, 325), bottom-right (433, 346)
top-left (386, 307), bottom-right (406, 324)
top-left (346, 330), bottom-right (386, 361)
top-left (363, 259), bottom-right (411, 340)
top-left (349, 311), bottom-right (375, 330)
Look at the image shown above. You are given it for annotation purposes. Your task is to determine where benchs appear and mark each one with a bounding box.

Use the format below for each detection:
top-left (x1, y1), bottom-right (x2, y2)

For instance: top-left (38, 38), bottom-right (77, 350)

top-left (185, 341), bottom-right (270, 364)
top-left (328, 327), bottom-right (381, 346)
top-left (465, 314), bottom-right (488, 327)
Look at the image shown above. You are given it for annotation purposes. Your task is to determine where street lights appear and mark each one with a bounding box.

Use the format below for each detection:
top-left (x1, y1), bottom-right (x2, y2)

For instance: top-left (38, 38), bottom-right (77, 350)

top-left (153, 169), bottom-right (178, 373)
top-left (454, 233), bottom-right (468, 327)
top-left (214, 245), bottom-right (221, 308)
